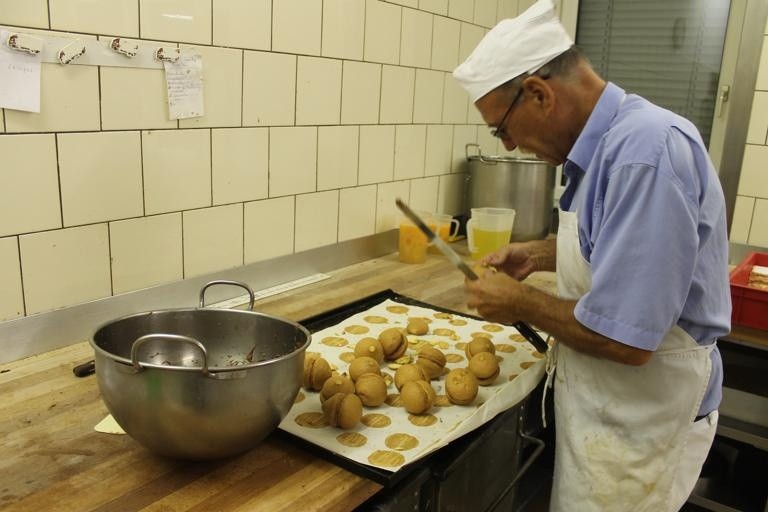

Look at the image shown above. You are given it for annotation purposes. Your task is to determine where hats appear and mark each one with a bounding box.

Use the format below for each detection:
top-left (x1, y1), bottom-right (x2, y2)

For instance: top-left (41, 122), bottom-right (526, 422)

top-left (452, 0), bottom-right (574, 104)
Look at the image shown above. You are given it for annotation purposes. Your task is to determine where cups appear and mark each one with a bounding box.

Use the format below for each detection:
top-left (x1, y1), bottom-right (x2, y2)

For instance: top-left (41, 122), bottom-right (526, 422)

top-left (465, 206), bottom-right (518, 261)
top-left (398, 210), bottom-right (441, 265)
top-left (430, 212), bottom-right (460, 255)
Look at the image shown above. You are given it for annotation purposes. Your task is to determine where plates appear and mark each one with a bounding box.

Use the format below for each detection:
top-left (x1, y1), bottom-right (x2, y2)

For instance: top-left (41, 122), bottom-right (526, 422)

top-left (272, 289), bottom-right (552, 487)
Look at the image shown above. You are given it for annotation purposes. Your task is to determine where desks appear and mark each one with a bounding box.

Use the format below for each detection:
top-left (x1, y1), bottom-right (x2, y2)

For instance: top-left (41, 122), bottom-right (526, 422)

top-left (1, 233), bottom-right (583, 512)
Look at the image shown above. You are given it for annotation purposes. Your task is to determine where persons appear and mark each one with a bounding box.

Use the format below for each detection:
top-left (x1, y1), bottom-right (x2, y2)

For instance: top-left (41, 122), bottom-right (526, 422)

top-left (452, 1), bottom-right (732, 510)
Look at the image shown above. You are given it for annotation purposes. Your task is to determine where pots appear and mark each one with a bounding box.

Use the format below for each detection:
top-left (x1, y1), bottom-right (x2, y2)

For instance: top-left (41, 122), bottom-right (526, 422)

top-left (460, 143), bottom-right (558, 243)
top-left (87, 278), bottom-right (314, 466)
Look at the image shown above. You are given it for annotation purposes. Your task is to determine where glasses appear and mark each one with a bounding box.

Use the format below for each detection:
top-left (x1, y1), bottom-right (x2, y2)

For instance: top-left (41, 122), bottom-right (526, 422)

top-left (489, 74), bottom-right (552, 142)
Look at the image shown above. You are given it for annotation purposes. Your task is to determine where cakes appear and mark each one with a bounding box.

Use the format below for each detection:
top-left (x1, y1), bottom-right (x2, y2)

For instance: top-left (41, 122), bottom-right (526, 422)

top-left (394, 364), bottom-right (431, 390)
top-left (469, 351), bottom-right (500, 386)
top-left (417, 347), bottom-right (445, 378)
top-left (407, 320), bottom-right (427, 335)
top-left (466, 337), bottom-right (495, 359)
top-left (400, 380), bottom-right (435, 415)
top-left (349, 356), bottom-right (381, 380)
top-left (302, 352), bottom-right (332, 391)
top-left (354, 338), bottom-right (383, 363)
top-left (378, 329), bottom-right (408, 360)
top-left (320, 375), bottom-right (354, 404)
top-left (355, 373), bottom-right (387, 407)
top-left (444, 368), bottom-right (478, 406)
top-left (321, 393), bottom-right (362, 429)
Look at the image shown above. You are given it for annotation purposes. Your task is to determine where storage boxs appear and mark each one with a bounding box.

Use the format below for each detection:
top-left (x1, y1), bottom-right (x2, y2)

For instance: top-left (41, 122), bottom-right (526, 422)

top-left (730, 251), bottom-right (768, 330)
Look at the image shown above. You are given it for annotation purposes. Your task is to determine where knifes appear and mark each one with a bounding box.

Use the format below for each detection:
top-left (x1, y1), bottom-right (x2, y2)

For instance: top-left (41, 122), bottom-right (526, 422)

top-left (394, 195), bottom-right (549, 359)
